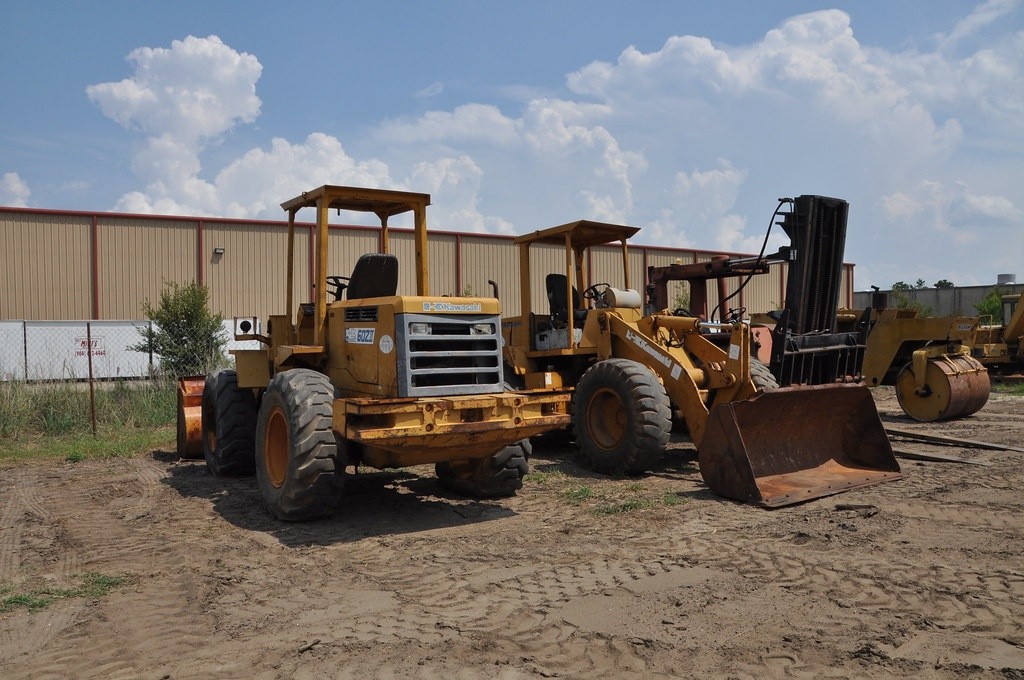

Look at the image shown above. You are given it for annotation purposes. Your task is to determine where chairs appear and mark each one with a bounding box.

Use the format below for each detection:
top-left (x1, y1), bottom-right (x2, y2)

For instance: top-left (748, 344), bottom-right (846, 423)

top-left (343, 251), bottom-right (400, 300)
top-left (546, 273), bottom-right (593, 328)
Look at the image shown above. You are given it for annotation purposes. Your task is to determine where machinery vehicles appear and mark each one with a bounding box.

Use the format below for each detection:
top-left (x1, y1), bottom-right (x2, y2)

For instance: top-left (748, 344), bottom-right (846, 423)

top-left (455, 190), bottom-right (902, 516)
top-left (173, 185), bottom-right (572, 507)
top-left (963, 293), bottom-right (1024, 383)
top-left (644, 197), bottom-right (994, 421)
